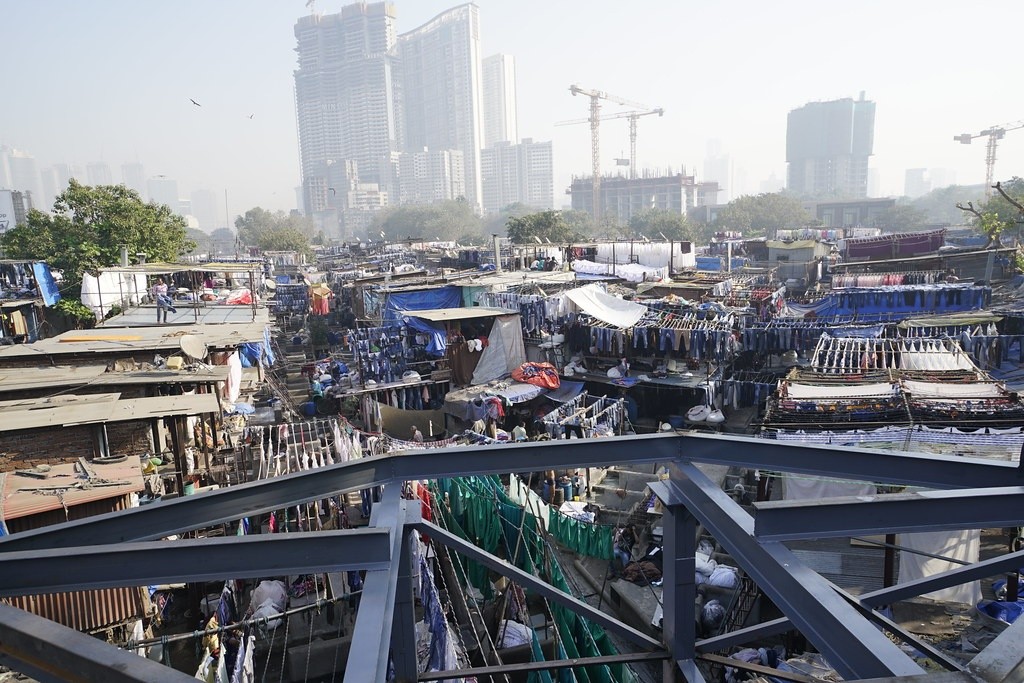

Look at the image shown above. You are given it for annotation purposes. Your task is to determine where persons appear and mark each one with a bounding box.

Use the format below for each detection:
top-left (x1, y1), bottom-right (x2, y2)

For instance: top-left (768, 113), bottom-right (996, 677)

top-left (153, 278), bottom-right (168, 323)
top-left (307, 376), bottom-right (321, 399)
top-left (530, 256), bottom-right (560, 271)
top-left (332, 362), bottom-right (340, 382)
top-left (408, 426), bottom-right (423, 443)
top-left (513, 422), bottom-right (526, 439)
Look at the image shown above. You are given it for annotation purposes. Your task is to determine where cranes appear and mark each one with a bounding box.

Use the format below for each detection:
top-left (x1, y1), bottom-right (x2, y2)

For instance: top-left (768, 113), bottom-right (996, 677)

top-left (952, 119), bottom-right (1024, 205)
top-left (552, 106), bottom-right (665, 179)
top-left (567, 81), bottom-right (650, 222)
